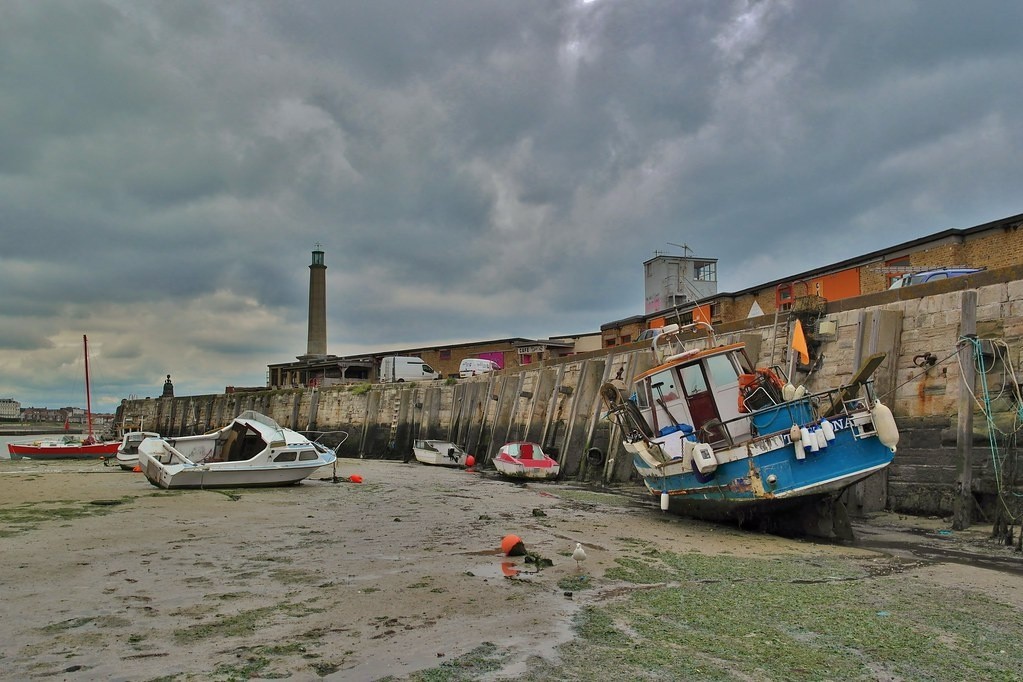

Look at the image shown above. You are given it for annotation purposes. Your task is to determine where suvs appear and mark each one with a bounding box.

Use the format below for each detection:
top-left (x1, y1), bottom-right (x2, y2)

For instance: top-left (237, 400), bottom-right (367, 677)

top-left (884, 267), bottom-right (990, 291)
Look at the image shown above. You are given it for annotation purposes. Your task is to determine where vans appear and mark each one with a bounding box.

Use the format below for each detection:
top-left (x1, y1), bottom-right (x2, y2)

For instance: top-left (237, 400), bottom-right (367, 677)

top-left (458, 358), bottom-right (502, 378)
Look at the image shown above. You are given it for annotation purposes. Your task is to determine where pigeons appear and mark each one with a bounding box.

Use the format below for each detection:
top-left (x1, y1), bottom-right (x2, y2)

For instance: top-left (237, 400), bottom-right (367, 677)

top-left (571, 543), bottom-right (587, 564)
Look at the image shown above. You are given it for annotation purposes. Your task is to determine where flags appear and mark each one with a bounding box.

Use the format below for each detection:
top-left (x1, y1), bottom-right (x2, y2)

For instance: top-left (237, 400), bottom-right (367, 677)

top-left (792, 319), bottom-right (809, 365)
top-left (65, 419), bottom-right (69, 430)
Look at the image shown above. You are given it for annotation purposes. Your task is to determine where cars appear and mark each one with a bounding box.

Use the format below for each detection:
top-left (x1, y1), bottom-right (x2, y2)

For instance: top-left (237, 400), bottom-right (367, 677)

top-left (632, 327), bottom-right (663, 342)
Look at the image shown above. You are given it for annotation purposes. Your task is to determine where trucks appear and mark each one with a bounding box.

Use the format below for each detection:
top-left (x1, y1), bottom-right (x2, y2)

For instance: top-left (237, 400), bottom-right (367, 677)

top-left (378, 356), bottom-right (439, 383)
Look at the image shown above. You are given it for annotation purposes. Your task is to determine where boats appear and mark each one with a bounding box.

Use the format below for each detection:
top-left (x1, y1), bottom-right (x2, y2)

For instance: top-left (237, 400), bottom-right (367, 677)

top-left (115, 415), bottom-right (350, 489)
top-left (491, 441), bottom-right (560, 480)
top-left (413, 438), bottom-right (468, 465)
top-left (599, 284), bottom-right (899, 524)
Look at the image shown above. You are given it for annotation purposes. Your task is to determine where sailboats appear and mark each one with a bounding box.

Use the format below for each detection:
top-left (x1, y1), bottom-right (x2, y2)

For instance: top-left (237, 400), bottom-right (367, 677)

top-left (7, 333), bottom-right (123, 459)
